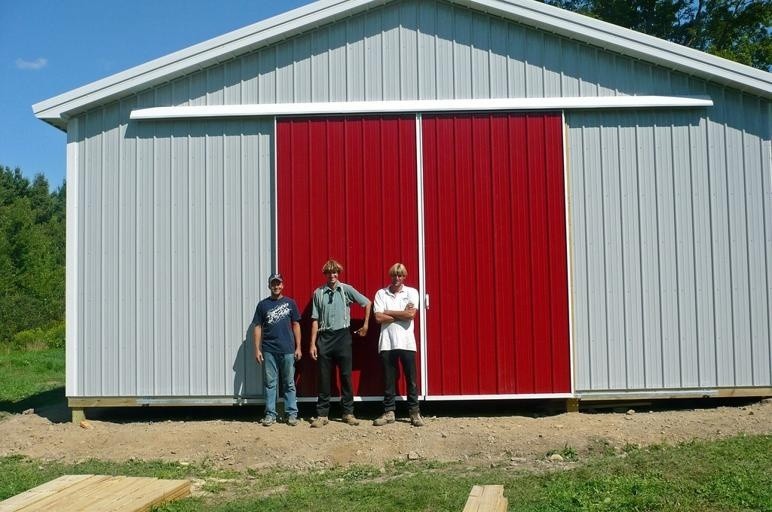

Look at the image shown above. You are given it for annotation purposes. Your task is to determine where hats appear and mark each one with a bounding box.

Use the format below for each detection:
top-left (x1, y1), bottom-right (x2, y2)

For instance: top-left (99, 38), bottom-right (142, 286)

top-left (268, 274), bottom-right (283, 283)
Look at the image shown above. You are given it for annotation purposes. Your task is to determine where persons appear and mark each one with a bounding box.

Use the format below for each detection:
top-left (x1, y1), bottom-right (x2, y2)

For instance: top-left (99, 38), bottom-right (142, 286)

top-left (251, 274), bottom-right (303, 427)
top-left (373, 263), bottom-right (426, 427)
top-left (309, 260), bottom-right (372, 428)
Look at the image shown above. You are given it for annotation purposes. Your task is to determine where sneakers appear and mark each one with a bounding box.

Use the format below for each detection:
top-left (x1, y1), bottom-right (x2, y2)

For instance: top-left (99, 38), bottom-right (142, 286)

top-left (411, 414), bottom-right (424, 426)
top-left (342, 416), bottom-right (359, 426)
top-left (285, 416), bottom-right (296, 426)
top-left (263, 415), bottom-right (275, 426)
top-left (374, 411), bottom-right (395, 426)
top-left (310, 417), bottom-right (327, 427)
